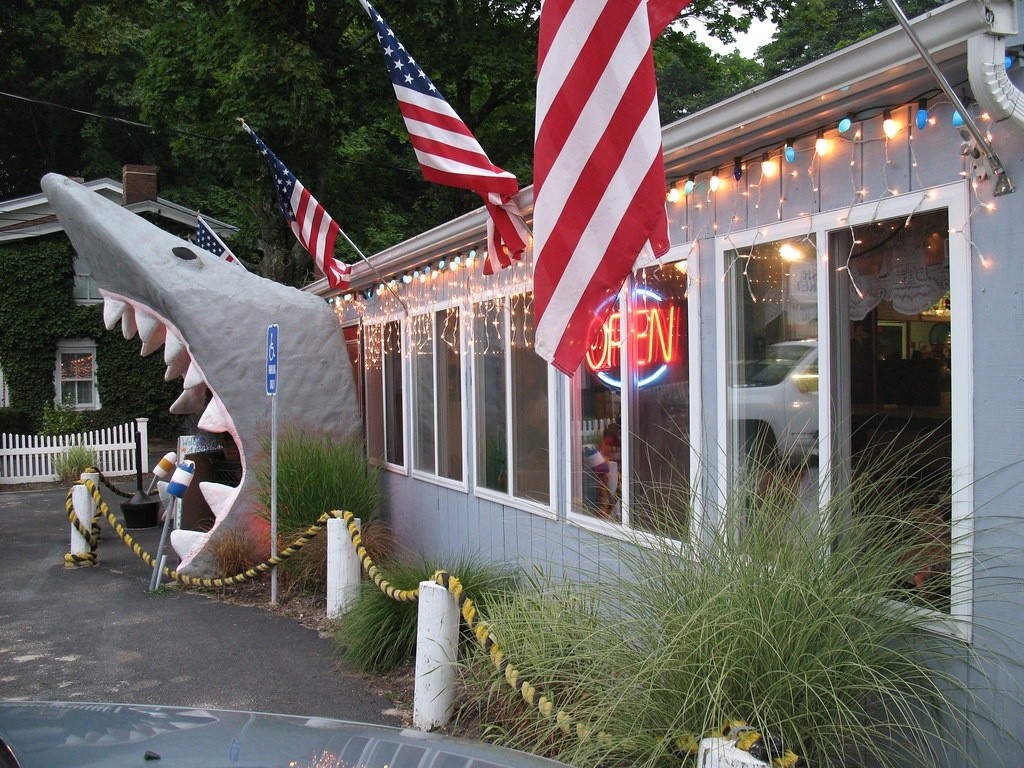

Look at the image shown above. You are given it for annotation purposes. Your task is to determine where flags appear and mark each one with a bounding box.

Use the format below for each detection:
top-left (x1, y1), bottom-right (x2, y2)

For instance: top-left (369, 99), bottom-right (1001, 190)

top-left (195, 218), bottom-right (249, 272)
top-left (360, 0), bottom-right (533, 276)
top-left (530, 0), bottom-right (693, 378)
top-left (240, 120), bottom-right (353, 292)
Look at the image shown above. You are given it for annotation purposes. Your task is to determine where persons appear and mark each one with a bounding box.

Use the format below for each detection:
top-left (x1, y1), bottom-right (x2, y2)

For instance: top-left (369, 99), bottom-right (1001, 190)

top-left (578, 419), bottom-right (620, 533)
top-left (212, 434), bottom-right (242, 489)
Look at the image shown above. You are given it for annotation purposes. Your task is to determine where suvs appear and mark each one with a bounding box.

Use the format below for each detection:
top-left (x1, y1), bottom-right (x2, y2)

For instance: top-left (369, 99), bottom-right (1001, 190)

top-left (639, 337), bottom-right (818, 482)
